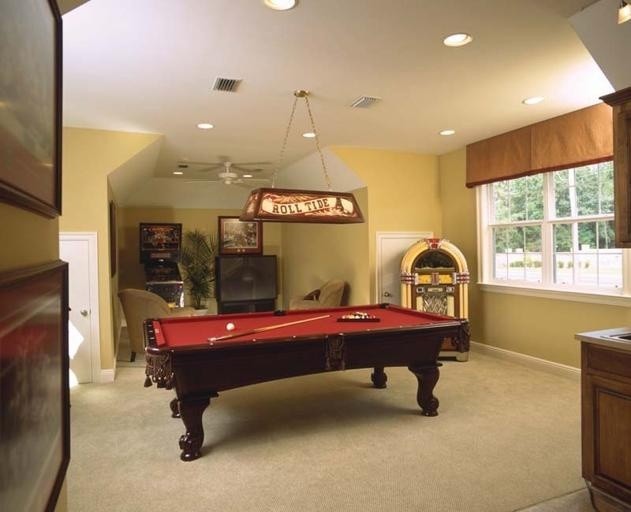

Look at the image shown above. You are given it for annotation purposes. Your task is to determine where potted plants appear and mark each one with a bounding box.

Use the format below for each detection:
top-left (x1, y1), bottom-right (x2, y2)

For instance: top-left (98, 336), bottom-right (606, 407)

top-left (176, 228), bottom-right (216, 316)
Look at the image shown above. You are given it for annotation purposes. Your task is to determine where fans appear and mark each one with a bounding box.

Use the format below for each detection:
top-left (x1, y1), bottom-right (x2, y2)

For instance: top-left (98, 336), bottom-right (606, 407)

top-left (185, 161), bottom-right (269, 185)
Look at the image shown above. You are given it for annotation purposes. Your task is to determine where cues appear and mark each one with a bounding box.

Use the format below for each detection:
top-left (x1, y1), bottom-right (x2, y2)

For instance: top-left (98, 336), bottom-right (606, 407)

top-left (207, 315), bottom-right (330, 341)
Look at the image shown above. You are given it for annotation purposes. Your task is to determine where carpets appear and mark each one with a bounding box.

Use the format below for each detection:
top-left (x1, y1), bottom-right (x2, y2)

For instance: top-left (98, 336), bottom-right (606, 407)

top-left (66, 342), bottom-right (593, 512)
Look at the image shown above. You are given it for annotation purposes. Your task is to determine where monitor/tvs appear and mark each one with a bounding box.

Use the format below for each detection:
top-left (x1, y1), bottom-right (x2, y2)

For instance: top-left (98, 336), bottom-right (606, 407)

top-left (215, 254), bottom-right (279, 303)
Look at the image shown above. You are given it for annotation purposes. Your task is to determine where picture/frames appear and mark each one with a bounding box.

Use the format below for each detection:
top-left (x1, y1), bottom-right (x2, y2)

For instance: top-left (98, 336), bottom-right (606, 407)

top-left (217, 216), bottom-right (263, 256)
top-left (0, 259), bottom-right (71, 512)
top-left (0, 0), bottom-right (63, 219)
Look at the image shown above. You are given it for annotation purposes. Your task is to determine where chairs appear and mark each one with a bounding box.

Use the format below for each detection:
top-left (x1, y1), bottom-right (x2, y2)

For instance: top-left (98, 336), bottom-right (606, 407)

top-left (288, 278), bottom-right (344, 311)
top-left (117, 288), bottom-right (208, 362)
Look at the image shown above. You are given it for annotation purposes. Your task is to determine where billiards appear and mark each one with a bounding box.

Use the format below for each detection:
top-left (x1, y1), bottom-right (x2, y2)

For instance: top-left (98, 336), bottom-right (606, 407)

top-left (227, 323), bottom-right (234, 331)
top-left (340, 312), bottom-right (375, 319)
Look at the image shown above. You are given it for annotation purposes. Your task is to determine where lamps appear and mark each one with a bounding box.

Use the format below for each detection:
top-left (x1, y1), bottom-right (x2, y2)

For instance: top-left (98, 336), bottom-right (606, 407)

top-left (239, 90), bottom-right (365, 225)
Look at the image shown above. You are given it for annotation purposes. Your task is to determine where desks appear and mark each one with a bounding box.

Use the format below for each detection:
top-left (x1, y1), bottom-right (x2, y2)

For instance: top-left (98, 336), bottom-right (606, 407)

top-left (575, 325), bottom-right (631, 512)
top-left (144, 303), bottom-right (469, 460)
